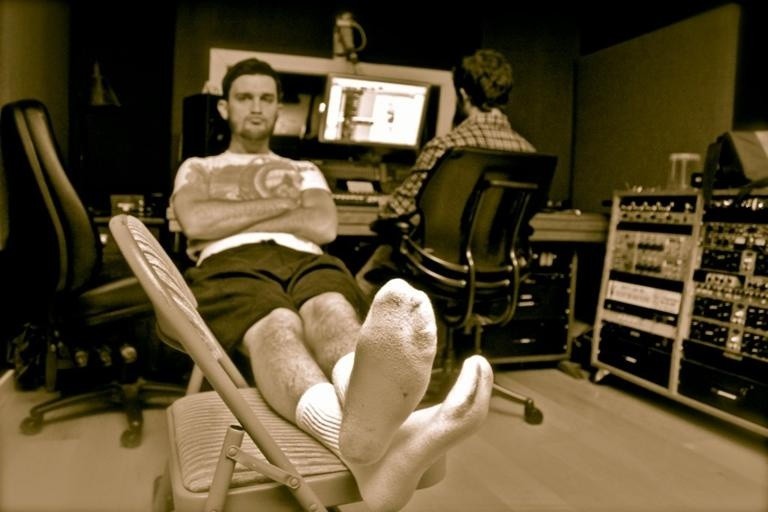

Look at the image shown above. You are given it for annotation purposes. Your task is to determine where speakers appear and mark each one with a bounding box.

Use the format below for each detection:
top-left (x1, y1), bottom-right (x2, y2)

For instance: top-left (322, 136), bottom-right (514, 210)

top-left (182, 94), bottom-right (231, 163)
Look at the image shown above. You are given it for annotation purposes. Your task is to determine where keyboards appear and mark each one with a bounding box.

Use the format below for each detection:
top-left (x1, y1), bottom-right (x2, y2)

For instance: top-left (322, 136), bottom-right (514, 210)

top-left (331, 194), bottom-right (395, 206)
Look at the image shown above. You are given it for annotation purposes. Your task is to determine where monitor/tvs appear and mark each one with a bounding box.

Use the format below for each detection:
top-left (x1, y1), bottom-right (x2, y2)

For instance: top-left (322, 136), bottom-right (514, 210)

top-left (318, 72), bottom-right (432, 164)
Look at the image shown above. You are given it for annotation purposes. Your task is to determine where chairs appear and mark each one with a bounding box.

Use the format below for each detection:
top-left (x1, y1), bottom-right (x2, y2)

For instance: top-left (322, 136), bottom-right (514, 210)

top-left (363, 144), bottom-right (557, 425)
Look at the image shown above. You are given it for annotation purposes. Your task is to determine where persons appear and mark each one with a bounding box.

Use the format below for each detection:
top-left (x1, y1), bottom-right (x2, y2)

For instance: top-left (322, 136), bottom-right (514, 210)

top-left (169, 57), bottom-right (495, 510)
top-left (354, 48), bottom-right (539, 377)
